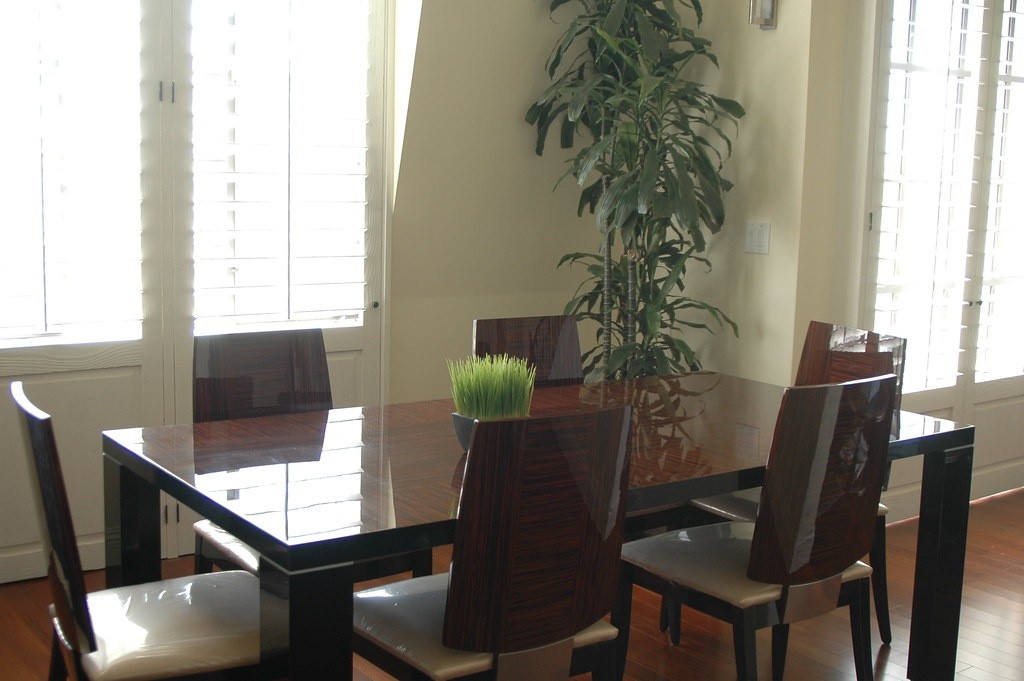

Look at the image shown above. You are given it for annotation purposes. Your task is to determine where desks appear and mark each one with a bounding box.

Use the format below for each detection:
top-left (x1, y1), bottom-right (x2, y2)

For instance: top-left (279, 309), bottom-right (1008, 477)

top-left (104, 372), bottom-right (977, 681)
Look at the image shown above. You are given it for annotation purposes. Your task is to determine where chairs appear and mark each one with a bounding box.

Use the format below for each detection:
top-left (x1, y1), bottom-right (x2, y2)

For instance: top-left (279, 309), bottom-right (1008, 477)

top-left (191, 326), bottom-right (433, 605)
top-left (615, 371), bottom-right (896, 681)
top-left (349, 406), bottom-right (635, 681)
top-left (656, 322), bottom-right (906, 644)
top-left (7, 378), bottom-right (259, 681)
top-left (473, 315), bottom-right (679, 633)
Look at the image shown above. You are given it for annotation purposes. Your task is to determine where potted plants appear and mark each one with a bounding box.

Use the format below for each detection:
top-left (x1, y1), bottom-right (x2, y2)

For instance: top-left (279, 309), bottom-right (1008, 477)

top-left (446, 353), bottom-right (537, 448)
